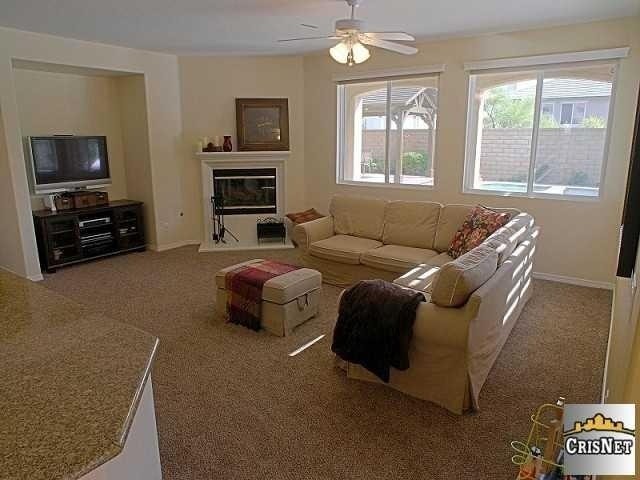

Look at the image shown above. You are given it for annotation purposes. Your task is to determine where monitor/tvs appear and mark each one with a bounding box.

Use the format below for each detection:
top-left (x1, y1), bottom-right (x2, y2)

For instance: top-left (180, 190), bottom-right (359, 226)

top-left (25, 134), bottom-right (112, 196)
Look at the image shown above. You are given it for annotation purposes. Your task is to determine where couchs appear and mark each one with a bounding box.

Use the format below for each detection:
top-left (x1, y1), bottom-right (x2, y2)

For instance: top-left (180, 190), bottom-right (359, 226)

top-left (284, 215), bottom-right (542, 416)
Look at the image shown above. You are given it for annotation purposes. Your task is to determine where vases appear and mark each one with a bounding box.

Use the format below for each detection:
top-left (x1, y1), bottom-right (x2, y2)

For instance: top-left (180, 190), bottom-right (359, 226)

top-left (223, 136), bottom-right (232, 152)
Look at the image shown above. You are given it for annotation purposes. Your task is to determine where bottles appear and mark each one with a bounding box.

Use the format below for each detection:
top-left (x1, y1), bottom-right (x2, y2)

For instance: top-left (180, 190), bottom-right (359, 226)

top-left (520, 445), bottom-right (543, 480)
top-left (223, 135), bottom-right (232, 152)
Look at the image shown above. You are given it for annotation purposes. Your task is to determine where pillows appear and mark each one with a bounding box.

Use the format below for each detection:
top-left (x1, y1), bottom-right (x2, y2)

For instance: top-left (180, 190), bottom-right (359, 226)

top-left (329, 194), bottom-right (535, 308)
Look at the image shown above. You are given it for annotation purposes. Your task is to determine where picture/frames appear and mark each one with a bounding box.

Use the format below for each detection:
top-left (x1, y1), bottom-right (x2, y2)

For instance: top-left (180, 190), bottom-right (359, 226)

top-left (235, 98), bottom-right (289, 151)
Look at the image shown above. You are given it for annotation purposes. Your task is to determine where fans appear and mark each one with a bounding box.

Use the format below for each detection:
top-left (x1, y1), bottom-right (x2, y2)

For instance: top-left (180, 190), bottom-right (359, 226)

top-left (277, 0), bottom-right (418, 55)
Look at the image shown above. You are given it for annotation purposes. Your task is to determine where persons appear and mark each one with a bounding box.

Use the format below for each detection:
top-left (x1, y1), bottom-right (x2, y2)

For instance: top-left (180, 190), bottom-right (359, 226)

top-left (510, 397), bottom-right (591, 480)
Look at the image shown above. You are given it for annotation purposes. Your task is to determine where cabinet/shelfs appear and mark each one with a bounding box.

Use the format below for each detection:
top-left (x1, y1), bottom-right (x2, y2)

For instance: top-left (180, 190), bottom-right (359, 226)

top-left (32, 199), bottom-right (147, 274)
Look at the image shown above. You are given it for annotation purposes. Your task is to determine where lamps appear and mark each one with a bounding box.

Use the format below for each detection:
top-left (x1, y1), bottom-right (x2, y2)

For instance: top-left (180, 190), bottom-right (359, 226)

top-left (328, 37), bottom-right (372, 66)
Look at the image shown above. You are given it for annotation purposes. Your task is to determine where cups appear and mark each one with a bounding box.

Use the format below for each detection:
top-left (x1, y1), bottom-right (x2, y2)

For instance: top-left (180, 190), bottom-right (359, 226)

top-left (199, 135), bottom-right (220, 149)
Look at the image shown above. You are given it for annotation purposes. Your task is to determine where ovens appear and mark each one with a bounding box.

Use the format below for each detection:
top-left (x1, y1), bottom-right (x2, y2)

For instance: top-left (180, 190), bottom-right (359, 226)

top-left (211, 167), bottom-right (278, 216)
